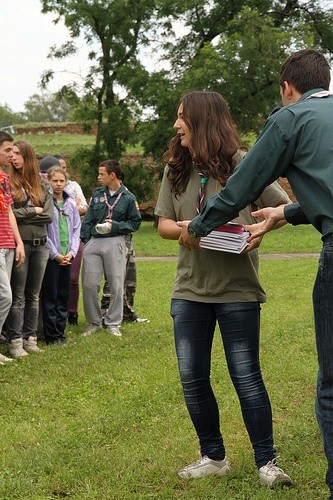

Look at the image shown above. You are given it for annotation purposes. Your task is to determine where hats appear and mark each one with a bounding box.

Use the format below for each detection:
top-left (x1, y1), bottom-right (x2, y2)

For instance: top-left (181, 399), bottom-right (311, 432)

top-left (40, 155), bottom-right (61, 172)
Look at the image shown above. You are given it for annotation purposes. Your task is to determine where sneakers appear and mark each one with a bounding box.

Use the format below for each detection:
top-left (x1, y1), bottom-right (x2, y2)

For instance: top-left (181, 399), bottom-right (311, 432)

top-left (257, 455), bottom-right (293, 489)
top-left (177, 455), bottom-right (230, 479)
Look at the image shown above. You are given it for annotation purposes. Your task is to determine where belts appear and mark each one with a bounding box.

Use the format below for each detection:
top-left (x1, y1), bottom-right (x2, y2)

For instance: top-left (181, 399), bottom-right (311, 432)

top-left (22, 236), bottom-right (46, 246)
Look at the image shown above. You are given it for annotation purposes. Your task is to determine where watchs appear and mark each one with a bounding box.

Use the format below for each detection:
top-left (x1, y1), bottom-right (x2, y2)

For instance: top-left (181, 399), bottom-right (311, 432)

top-left (188, 223), bottom-right (198, 239)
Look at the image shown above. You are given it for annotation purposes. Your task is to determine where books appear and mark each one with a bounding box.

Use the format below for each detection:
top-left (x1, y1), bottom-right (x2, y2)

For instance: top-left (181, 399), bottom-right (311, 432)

top-left (199, 222), bottom-right (251, 255)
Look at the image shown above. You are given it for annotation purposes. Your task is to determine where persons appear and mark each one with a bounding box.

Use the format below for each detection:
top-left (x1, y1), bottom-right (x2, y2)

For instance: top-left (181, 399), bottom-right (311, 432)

top-left (57, 159), bottom-right (89, 325)
top-left (77, 160), bottom-right (151, 340)
top-left (154, 91), bottom-right (294, 488)
top-left (175, 49), bottom-right (333, 500)
top-left (46, 165), bottom-right (82, 343)
top-left (10, 141), bottom-right (55, 359)
top-left (0, 131), bottom-right (26, 367)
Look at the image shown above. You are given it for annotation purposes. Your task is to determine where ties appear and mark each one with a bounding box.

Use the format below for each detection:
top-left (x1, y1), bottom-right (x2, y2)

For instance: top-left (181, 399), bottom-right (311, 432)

top-left (53, 199), bottom-right (69, 223)
top-left (103, 192), bottom-right (122, 220)
top-left (190, 154), bottom-right (211, 216)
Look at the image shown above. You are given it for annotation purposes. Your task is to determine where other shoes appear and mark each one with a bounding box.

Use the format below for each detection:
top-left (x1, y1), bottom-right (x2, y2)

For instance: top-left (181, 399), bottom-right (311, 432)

top-left (80, 322), bottom-right (102, 337)
top-left (58, 336), bottom-right (67, 345)
top-left (49, 337), bottom-right (62, 346)
top-left (0, 353), bottom-right (16, 365)
top-left (105, 324), bottom-right (122, 337)
top-left (6, 337), bottom-right (28, 357)
top-left (23, 336), bottom-right (44, 353)
top-left (132, 317), bottom-right (150, 323)
